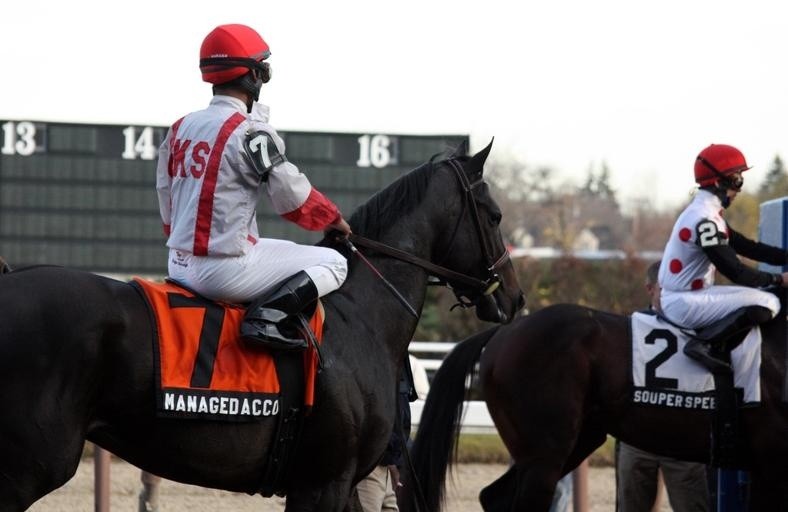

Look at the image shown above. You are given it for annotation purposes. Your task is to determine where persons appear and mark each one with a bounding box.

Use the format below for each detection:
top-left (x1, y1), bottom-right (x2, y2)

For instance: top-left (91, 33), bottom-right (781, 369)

top-left (153, 22), bottom-right (353, 351)
top-left (506, 452), bottom-right (588, 512)
top-left (654, 142), bottom-right (788, 376)
top-left (353, 360), bottom-right (414, 511)
top-left (610, 439), bottom-right (710, 512)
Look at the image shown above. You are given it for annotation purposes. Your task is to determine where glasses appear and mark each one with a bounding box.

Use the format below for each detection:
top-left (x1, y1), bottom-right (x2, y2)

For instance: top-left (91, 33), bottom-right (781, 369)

top-left (260, 59), bottom-right (272, 82)
top-left (727, 175), bottom-right (744, 191)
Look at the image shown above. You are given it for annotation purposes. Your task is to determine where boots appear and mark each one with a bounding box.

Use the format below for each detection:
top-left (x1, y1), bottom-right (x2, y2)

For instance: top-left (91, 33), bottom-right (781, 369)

top-left (235, 268), bottom-right (322, 354)
top-left (681, 303), bottom-right (773, 377)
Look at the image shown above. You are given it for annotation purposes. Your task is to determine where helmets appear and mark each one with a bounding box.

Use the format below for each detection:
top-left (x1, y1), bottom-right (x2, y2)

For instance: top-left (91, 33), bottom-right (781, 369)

top-left (199, 22), bottom-right (272, 86)
top-left (693, 141), bottom-right (753, 190)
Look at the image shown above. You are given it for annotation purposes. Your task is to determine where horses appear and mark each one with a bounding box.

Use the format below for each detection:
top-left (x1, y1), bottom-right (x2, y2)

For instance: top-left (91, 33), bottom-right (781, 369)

top-left (396, 283), bottom-right (788, 512)
top-left (0, 135), bottom-right (526, 512)
